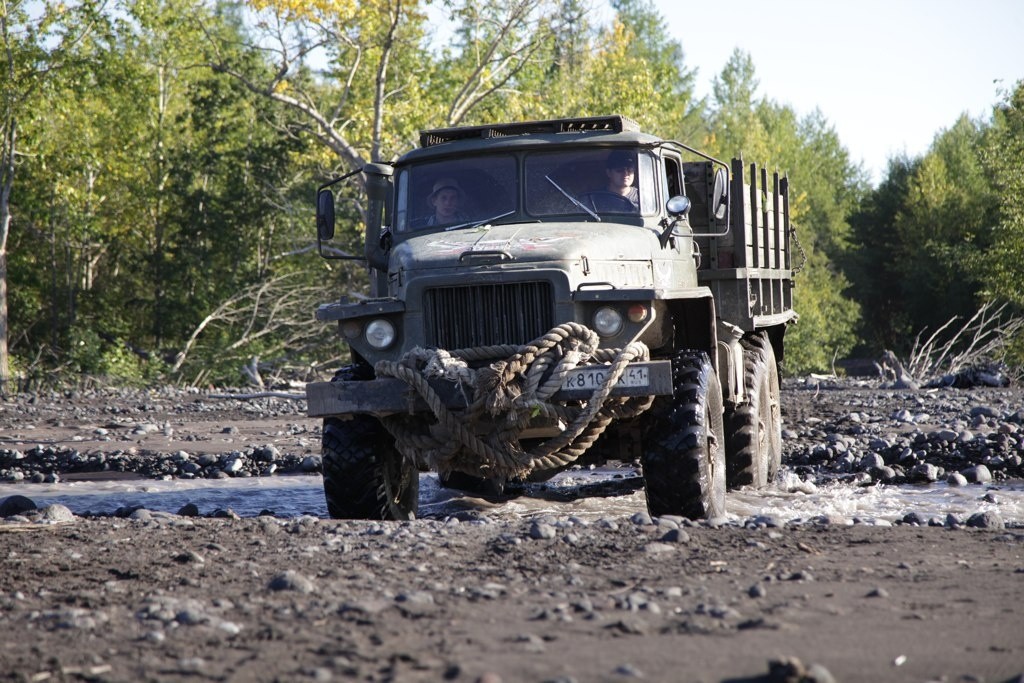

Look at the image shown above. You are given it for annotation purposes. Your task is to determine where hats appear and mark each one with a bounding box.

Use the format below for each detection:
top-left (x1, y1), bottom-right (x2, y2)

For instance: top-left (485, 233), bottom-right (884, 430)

top-left (427, 178), bottom-right (465, 210)
top-left (607, 151), bottom-right (638, 170)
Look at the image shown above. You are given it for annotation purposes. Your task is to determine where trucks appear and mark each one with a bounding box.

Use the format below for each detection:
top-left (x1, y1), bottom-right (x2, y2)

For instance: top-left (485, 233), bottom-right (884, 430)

top-left (305, 116), bottom-right (797, 523)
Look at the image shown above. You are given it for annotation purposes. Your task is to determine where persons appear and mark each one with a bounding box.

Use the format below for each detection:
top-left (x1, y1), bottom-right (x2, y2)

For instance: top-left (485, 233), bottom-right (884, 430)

top-left (564, 150), bottom-right (640, 214)
top-left (407, 176), bottom-right (473, 229)
